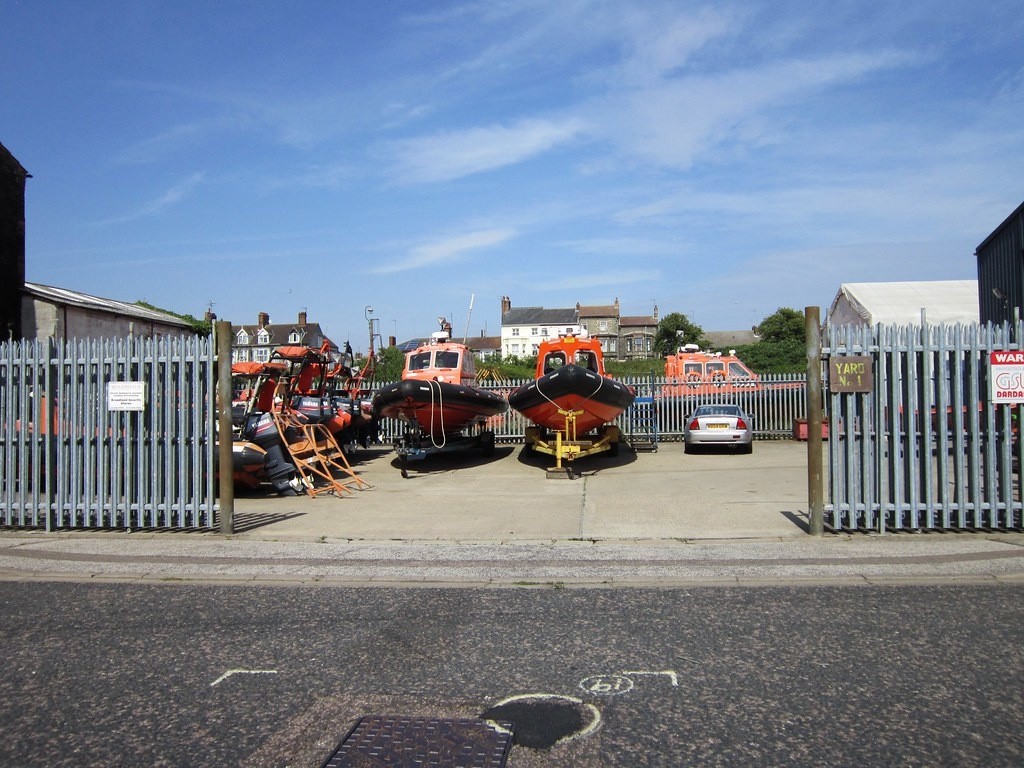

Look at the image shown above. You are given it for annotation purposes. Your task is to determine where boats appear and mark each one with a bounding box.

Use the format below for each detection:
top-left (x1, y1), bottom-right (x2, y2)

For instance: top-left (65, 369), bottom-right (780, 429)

top-left (507, 333), bottom-right (637, 440)
top-left (145, 337), bottom-right (376, 496)
top-left (370, 330), bottom-right (510, 438)
top-left (654, 343), bottom-right (762, 398)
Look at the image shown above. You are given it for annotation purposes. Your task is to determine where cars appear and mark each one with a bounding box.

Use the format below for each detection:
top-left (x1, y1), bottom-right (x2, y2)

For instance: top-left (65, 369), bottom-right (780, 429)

top-left (684, 403), bottom-right (755, 453)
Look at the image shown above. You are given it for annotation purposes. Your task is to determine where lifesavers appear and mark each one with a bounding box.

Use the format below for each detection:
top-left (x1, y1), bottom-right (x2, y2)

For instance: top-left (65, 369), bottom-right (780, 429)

top-left (708, 370), bottom-right (728, 389)
top-left (684, 371), bottom-right (702, 389)
